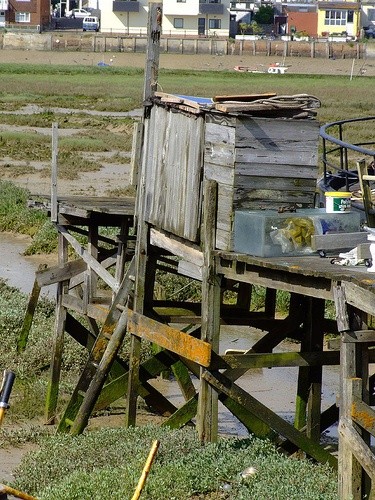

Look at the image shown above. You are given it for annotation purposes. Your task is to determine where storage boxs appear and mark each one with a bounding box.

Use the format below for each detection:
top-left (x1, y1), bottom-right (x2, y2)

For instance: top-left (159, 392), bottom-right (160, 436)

top-left (232, 209), bottom-right (362, 257)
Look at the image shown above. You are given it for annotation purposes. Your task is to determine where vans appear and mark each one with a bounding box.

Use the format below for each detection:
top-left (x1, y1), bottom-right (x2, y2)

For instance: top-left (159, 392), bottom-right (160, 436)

top-left (83, 16), bottom-right (99, 32)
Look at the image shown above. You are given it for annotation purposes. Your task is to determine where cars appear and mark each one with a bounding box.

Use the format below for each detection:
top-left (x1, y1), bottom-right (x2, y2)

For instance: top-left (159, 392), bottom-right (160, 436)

top-left (327, 32), bottom-right (353, 42)
top-left (66, 9), bottom-right (91, 19)
